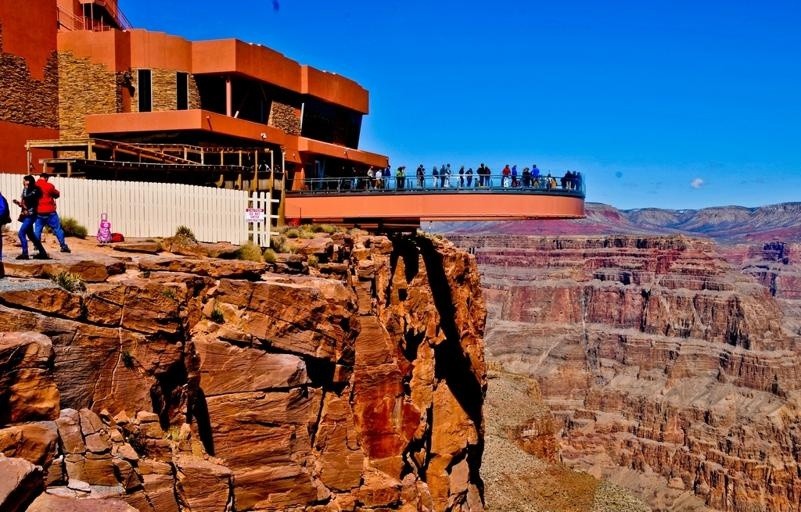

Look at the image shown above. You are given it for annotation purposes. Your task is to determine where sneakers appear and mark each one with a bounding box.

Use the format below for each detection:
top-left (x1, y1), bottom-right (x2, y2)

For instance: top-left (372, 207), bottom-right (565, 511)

top-left (16, 254), bottom-right (29, 260)
top-left (60, 245), bottom-right (71, 252)
top-left (33, 253), bottom-right (48, 258)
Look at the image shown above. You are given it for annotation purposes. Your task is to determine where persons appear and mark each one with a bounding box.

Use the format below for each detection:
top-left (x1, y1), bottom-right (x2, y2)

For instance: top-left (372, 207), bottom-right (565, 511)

top-left (1, 192), bottom-right (12, 278)
top-left (335, 162), bottom-right (583, 194)
top-left (33, 173), bottom-right (71, 252)
top-left (15, 175), bottom-right (49, 260)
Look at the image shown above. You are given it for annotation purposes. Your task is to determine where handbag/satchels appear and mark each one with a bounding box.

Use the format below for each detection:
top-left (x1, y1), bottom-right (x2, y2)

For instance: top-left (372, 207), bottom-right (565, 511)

top-left (111, 233), bottom-right (125, 242)
